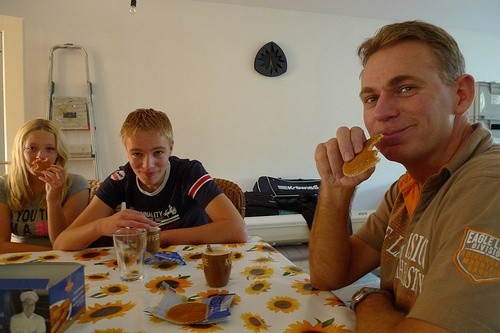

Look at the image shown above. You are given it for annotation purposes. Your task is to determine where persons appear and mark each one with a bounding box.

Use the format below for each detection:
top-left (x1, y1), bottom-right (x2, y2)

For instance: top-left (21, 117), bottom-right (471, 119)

top-left (53, 107), bottom-right (249, 252)
top-left (0, 118), bottom-right (90, 255)
top-left (9, 290), bottom-right (46, 333)
top-left (307, 21), bottom-right (500, 333)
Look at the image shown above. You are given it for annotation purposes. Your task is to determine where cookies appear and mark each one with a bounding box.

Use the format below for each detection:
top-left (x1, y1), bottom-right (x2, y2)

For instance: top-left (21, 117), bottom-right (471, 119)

top-left (32, 158), bottom-right (51, 173)
top-left (166, 302), bottom-right (209, 324)
top-left (343, 134), bottom-right (384, 177)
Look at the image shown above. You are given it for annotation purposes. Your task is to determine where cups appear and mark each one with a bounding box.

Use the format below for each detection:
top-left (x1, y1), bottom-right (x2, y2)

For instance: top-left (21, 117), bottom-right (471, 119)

top-left (113, 228), bottom-right (147, 281)
top-left (201, 246), bottom-right (232, 288)
top-left (145, 228), bottom-right (161, 253)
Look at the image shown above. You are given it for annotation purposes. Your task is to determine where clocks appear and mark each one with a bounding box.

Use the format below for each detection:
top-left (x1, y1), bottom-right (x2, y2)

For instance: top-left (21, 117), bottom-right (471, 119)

top-left (254, 41), bottom-right (288, 77)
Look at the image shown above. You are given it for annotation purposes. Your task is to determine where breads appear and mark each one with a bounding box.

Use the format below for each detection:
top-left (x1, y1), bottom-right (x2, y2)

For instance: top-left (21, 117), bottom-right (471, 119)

top-left (48, 298), bottom-right (70, 333)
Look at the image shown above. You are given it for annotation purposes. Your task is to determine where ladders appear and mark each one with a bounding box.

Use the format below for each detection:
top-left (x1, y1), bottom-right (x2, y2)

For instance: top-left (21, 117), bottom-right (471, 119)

top-left (45, 42), bottom-right (100, 181)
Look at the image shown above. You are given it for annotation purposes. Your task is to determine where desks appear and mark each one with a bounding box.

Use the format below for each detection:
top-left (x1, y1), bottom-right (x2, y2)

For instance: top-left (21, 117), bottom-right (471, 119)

top-left (0, 241), bottom-right (357, 333)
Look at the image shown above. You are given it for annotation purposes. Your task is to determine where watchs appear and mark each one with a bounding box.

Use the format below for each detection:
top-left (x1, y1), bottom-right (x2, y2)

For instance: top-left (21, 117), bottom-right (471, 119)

top-left (349, 287), bottom-right (395, 311)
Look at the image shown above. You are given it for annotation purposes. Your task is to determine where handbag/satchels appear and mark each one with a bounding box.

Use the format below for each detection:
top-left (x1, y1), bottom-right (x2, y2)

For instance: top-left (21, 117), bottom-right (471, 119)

top-left (253, 175), bottom-right (322, 206)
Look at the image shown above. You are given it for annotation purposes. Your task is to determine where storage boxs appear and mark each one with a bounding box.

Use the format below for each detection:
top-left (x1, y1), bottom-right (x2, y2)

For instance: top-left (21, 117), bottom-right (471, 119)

top-left (0, 262), bottom-right (85, 333)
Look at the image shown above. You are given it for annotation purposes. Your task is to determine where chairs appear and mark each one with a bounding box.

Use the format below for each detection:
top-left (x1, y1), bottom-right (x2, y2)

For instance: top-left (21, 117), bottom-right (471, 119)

top-left (208, 178), bottom-right (245, 223)
